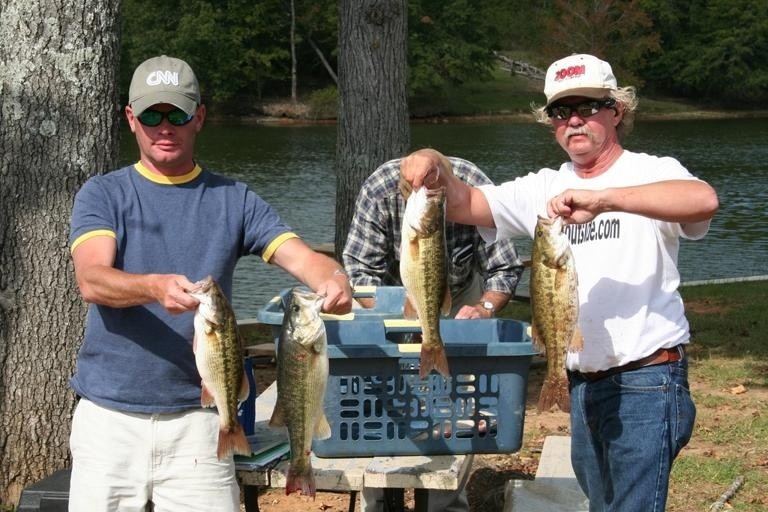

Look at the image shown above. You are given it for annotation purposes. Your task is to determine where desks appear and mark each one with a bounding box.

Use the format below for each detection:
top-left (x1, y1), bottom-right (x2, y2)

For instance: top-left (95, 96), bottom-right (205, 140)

top-left (236, 379), bottom-right (474, 511)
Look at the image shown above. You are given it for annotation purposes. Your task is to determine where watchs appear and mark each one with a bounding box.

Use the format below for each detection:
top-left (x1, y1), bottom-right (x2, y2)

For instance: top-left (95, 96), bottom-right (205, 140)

top-left (475, 300), bottom-right (496, 318)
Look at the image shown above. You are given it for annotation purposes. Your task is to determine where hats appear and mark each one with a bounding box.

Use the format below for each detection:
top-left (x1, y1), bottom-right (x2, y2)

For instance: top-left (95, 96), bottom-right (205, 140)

top-left (128, 55), bottom-right (201, 118)
top-left (543, 53), bottom-right (618, 104)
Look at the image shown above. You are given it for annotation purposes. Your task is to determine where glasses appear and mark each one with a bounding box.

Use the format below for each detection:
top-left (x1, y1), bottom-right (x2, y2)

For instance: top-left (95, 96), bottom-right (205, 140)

top-left (137, 108), bottom-right (192, 126)
top-left (546, 98), bottom-right (615, 120)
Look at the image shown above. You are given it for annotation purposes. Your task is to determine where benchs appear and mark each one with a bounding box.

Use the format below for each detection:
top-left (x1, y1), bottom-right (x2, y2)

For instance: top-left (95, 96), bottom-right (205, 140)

top-left (535, 433), bottom-right (577, 489)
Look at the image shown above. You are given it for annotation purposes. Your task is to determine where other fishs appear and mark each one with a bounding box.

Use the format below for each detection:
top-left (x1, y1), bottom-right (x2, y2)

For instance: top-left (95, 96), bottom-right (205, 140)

top-left (267, 287), bottom-right (332, 502)
top-left (414, 410), bottom-right (497, 440)
top-left (188, 274), bottom-right (250, 462)
top-left (398, 185), bottom-right (453, 380)
top-left (529, 213), bottom-right (585, 416)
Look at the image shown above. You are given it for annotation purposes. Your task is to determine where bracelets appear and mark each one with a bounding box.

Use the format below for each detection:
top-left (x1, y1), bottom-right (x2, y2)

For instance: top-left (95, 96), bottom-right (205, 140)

top-left (332, 269), bottom-right (350, 278)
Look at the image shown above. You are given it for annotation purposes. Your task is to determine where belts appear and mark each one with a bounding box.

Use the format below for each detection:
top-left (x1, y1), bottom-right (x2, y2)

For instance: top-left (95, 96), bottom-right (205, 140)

top-left (585, 347), bottom-right (680, 383)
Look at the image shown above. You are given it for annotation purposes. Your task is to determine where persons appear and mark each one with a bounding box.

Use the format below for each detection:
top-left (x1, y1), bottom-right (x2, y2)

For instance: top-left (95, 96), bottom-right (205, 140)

top-left (68, 54), bottom-right (355, 512)
top-left (342, 147), bottom-right (526, 320)
top-left (400, 53), bottom-right (720, 512)
